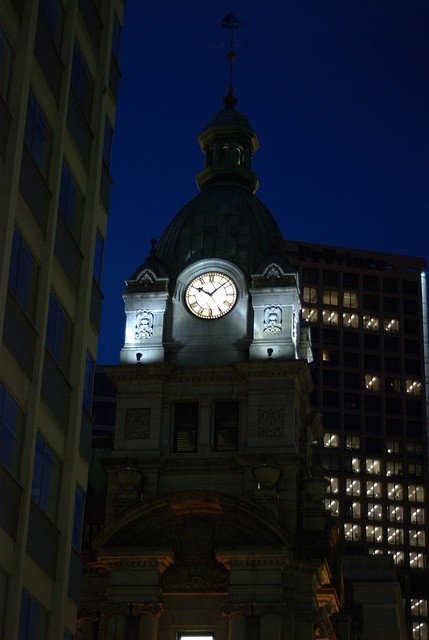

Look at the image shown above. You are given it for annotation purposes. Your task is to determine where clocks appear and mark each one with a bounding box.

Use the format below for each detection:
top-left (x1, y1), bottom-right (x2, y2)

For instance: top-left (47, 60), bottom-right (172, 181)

top-left (184, 271), bottom-right (239, 321)
